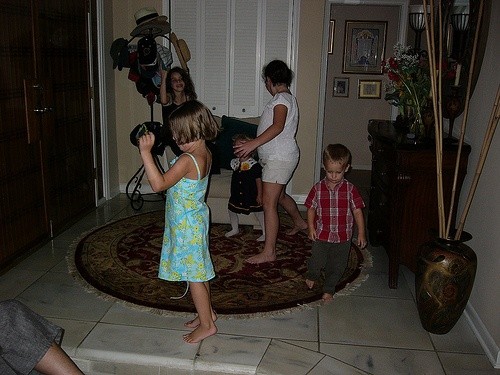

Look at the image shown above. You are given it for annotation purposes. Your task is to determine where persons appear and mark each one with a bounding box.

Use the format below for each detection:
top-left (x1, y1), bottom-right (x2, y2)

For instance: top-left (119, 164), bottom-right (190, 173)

top-left (225, 135), bottom-right (267, 242)
top-left (306, 144), bottom-right (367, 302)
top-left (233, 60), bottom-right (308, 264)
top-left (159, 64), bottom-right (198, 168)
top-left (0, 298), bottom-right (85, 375)
top-left (139, 99), bottom-right (217, 342)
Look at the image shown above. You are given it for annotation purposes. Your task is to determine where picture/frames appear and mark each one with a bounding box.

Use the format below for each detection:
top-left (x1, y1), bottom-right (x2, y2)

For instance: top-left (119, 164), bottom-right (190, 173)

top-left (342, 20), bottom-right (388, 75)
top-left (332, 77), bottom-right (350, 97)
top-left (328, 19), bottom-right (336, 54)
top-left (357, 80), bottom-right (382, 99)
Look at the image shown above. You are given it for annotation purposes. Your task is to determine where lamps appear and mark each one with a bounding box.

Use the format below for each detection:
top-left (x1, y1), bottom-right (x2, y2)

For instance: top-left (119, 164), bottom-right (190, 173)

top-left (450, 0), bottom-right (472, 59)
top-left (408, 5), bottom-right (432, 54)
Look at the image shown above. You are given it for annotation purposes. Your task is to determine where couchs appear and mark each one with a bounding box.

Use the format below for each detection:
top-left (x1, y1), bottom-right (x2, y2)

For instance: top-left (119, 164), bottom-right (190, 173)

top-left (205, 115), bottom-right (262, 230)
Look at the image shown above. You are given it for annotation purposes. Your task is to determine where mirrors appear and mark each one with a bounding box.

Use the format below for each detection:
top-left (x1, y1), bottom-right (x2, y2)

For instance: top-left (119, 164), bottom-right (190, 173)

top-left (435, 0), bottom-right (491, 118)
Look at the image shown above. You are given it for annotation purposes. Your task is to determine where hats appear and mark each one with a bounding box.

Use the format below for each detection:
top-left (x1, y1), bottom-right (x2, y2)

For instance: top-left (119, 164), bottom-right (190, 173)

top-left (110, 7), bottom-right (190, 105)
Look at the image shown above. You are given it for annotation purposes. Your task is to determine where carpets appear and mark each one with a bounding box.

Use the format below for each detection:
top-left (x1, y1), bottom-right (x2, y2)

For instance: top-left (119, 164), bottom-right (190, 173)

top-left (64, 207), bottom-right (375, 321)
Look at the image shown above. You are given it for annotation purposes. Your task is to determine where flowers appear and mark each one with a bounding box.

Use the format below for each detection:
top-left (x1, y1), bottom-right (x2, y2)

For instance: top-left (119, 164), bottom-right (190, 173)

top-left (381, 42), bottom-right (455, 136)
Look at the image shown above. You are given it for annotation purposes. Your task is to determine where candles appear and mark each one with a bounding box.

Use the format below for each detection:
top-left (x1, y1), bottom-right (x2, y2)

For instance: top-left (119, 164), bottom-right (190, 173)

top-left (455, 63), bottom-right (462, 86)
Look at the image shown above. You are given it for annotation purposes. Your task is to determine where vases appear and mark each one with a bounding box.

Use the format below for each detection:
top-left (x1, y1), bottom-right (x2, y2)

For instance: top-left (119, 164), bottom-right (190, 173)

top-left (402, 96), bottom-right (435, 144)
top-left (416, 228), bottom-right (478, 334)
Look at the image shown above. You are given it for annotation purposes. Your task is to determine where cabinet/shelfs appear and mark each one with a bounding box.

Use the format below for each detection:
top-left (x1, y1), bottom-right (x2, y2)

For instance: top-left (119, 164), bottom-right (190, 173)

top-left (367, 120), bottom-right (471, 290)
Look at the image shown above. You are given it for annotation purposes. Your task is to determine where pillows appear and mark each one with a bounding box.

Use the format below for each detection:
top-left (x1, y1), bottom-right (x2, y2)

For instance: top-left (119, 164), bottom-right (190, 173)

top-left (215, 115), bottom-right (258, 171)
top-left (168, 139), bottom-right (221, 174)
